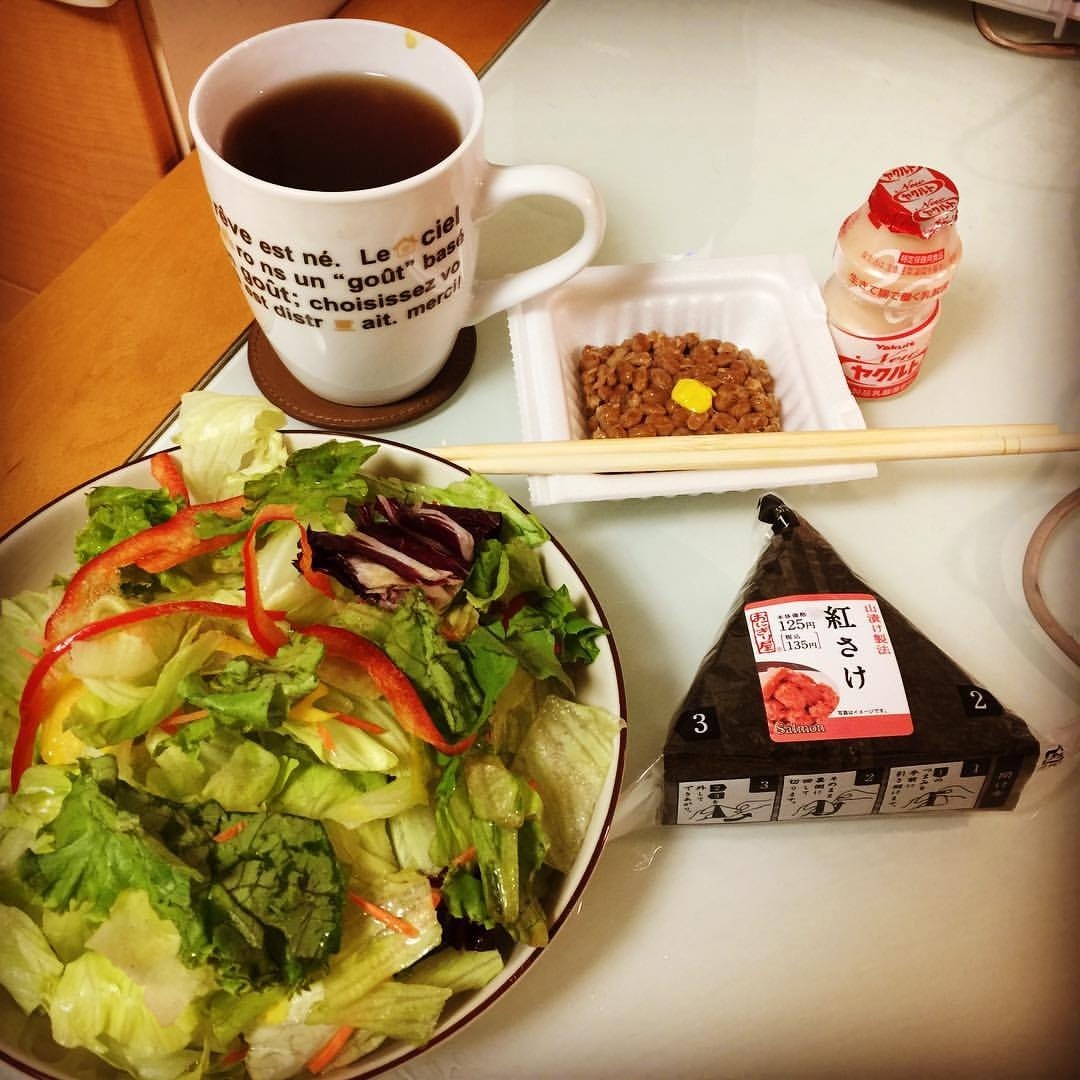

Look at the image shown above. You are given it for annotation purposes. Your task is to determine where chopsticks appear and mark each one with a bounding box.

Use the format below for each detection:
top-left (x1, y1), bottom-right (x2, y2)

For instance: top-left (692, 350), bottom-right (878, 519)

top-left (428, 421), bottom-right (1080, 475)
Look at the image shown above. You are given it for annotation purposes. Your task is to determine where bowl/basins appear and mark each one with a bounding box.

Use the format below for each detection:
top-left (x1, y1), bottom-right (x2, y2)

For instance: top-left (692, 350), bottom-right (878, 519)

top-left (503, 247), bottom-right (881, 508)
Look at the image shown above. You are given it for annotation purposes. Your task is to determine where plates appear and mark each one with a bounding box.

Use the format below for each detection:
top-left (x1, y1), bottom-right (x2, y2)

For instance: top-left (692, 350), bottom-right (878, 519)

top-left (0, 432), bottom-right (629, 1078)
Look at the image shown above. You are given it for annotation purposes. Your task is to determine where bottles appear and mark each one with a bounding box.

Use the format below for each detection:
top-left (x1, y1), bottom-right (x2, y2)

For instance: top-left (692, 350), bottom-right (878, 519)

top-left (821, 165), bottom-right (962, 401)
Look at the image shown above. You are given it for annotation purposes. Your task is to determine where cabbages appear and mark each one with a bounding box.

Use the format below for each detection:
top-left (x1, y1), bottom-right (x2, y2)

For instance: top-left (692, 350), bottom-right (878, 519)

top-left (0, 389), bottom-right (628, 1080)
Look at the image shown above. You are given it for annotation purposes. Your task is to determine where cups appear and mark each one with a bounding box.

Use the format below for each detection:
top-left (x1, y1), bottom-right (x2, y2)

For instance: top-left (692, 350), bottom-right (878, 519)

top-left (189, 17), bottom-right (609, 409)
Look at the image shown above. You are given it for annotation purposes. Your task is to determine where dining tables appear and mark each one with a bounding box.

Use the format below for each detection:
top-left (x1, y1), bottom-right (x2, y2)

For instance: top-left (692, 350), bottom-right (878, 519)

top-left (0, 1), bottom-right (1080, 1079)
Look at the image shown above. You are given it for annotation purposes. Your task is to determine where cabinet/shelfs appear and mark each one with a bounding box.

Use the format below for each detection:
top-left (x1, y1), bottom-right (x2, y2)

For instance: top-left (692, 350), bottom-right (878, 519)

top-left (2, 1), bottom-right (343, 330)
